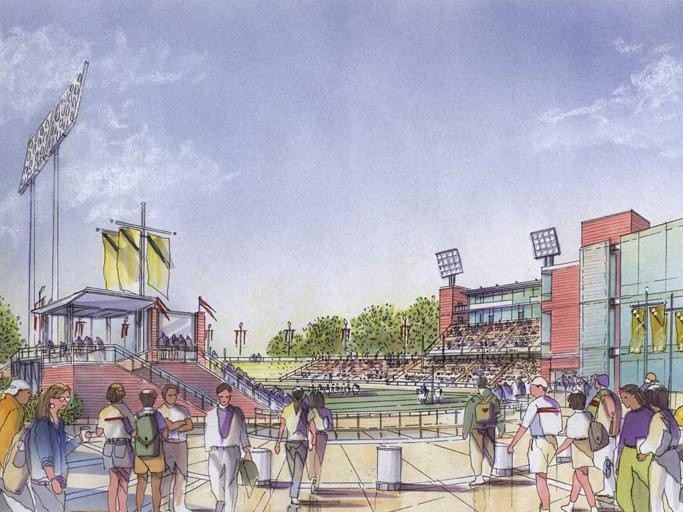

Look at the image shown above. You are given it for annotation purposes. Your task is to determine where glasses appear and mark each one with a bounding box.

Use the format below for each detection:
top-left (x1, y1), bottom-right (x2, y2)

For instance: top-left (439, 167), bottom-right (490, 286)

top-left (51, 396), bottom-right (71, 402)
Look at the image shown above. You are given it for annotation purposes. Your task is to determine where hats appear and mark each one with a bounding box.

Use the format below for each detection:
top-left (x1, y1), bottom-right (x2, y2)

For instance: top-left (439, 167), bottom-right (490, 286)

top-left (3, 379), bottom-right (30, 396)
top-left (594, 373), bottom-right (609, 387)
top-left (645, 372), bottom-right (660, 383)
top-left (526, 376), bottom-right (549, 388)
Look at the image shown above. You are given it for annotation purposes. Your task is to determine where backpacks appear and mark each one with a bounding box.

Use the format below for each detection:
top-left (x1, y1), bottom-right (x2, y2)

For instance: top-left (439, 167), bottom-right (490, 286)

top-left (134, 409), bottom-right (160, 457)
top-left (471, 392), bottom-right (495, 424)
top-left (602, 390), bottom-right (622, 438)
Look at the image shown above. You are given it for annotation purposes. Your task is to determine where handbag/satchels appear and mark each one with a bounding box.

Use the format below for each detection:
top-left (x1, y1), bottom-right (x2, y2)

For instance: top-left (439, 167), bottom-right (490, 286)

top-left (1, 428), bottom-right (30, 495)
top-left (588, 420), bottom-right (609, 452)
top-left (240, 458), bottom-right (261, 498)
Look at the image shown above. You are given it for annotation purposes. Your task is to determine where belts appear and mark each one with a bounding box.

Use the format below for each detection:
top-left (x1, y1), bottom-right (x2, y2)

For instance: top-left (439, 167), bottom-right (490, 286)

top-left (532, 434), bottom-right (556, 439)
